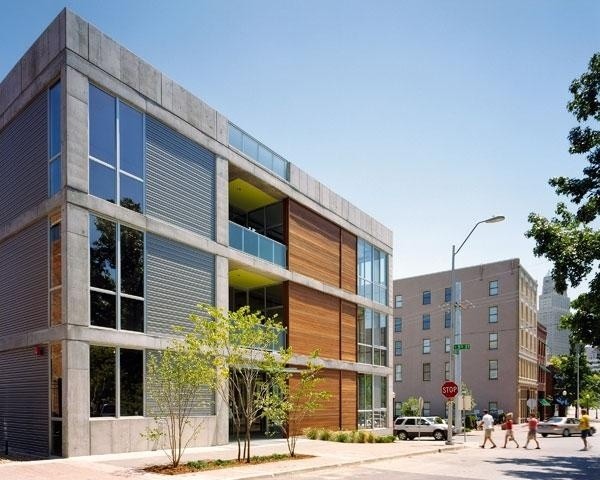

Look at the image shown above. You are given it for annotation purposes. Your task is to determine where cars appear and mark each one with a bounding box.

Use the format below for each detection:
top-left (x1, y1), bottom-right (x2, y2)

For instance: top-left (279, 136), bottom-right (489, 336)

top-left (536, 417), bottom-right (596, 437)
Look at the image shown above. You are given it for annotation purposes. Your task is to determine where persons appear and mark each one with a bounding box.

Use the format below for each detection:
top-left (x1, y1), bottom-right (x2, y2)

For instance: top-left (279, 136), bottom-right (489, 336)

top-left (522, 413), bottom-right (540, 449)
top-left (502, 412), bottom-right (519, 448)
top-left (476, 409), bottom-right (496, 449)
top-left (575, 409), bottom-right (589, 451)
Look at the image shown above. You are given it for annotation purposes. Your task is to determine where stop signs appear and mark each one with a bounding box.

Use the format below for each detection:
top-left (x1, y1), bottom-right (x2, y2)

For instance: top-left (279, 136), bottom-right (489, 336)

top-left (441, 381), bottom-right (459, 399)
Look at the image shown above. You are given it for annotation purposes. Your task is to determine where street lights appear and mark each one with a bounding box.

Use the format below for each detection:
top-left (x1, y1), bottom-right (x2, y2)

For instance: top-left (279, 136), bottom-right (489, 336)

top-left (586, 395), bottom-right (589, 415)
top-left (563, 391), bottom-right (567, 417)
top-left (445, 215), bottom-right (505, 445)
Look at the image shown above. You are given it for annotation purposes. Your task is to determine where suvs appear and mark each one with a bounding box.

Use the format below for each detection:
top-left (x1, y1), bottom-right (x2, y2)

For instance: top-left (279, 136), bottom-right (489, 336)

top-left (394, 416), bottom-right (456, 441)
